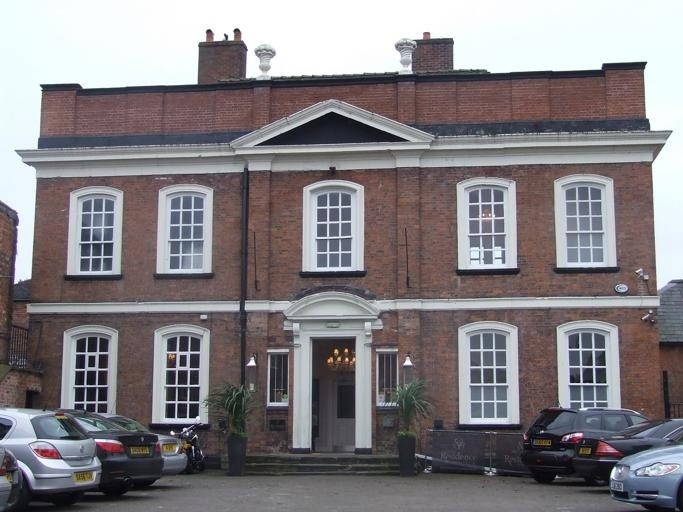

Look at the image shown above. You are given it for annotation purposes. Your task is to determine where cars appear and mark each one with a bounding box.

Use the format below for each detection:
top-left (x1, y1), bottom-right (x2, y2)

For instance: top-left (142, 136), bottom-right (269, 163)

top-left (0, 408), bottom-right (189, 512)
top-left (572, 416), bottom-right (683, 486)
top-left (520, 404), bottom-right (651, 487)
top-left (607, 443), bottom-right (683, 512)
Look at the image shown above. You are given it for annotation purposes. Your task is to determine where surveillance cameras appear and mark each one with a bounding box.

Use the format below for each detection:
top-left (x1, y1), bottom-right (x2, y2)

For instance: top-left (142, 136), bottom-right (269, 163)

top-left (641, 314), bottom-right (649, 321)
top-left (635, 268), bottom-right (642, 274)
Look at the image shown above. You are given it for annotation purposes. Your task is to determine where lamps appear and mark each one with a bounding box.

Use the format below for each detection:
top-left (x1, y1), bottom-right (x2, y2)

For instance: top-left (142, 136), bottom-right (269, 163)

top-left (326, 348), bottom-right (355, 372)
top-left (245, 351), bottom-right (258, 367)
top-left (402, 351), bottom-right (413, 368)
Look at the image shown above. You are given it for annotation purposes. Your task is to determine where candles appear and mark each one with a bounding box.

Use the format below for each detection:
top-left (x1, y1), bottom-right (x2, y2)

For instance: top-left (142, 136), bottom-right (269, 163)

top-left (382, 377), bottom-right (435, 476)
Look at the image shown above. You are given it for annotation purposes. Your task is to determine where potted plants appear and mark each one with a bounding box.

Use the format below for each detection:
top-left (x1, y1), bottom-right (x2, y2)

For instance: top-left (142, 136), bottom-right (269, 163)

top-left (201, 380), bottom-right (260, 474)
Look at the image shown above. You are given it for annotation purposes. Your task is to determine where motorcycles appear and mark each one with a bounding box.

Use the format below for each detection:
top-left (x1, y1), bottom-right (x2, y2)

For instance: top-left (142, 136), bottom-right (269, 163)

top-left (169, 414), bottom-right (208, 475)
